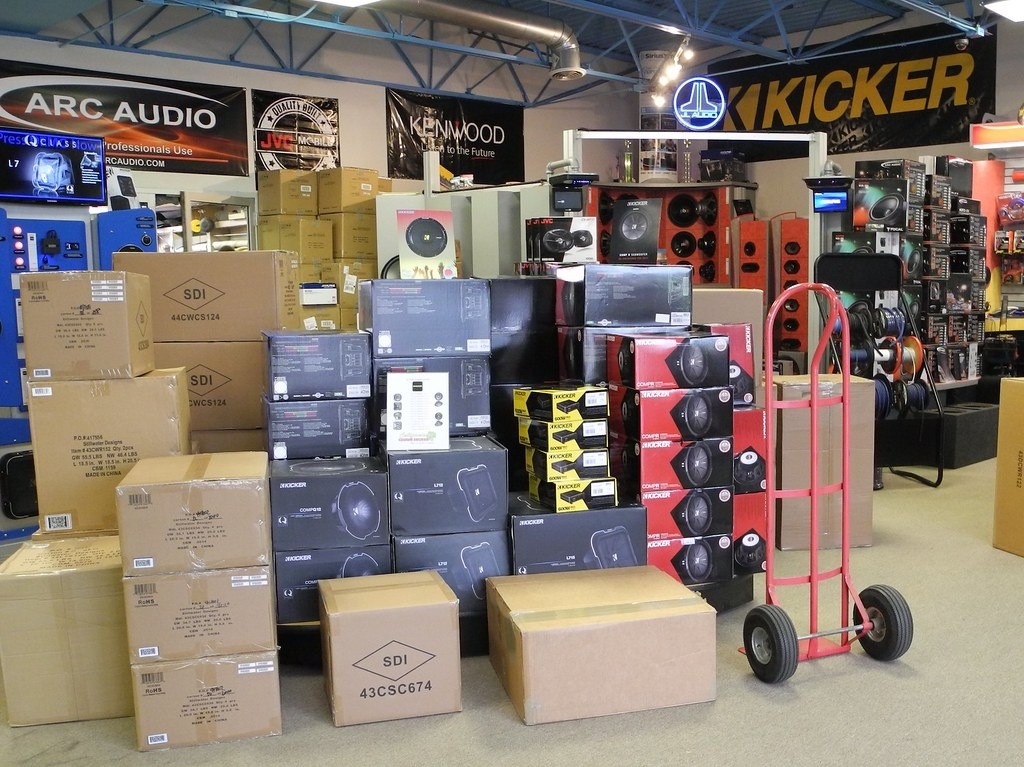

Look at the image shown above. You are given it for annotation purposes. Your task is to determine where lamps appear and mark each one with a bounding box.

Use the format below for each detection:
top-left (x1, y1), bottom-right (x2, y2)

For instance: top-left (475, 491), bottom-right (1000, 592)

top-left (647, 34), bottom-right (694, 111)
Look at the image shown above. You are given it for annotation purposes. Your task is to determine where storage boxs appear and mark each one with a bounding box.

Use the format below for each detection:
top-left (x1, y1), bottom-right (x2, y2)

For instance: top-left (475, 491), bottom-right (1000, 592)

top-left (853, 158), bottom-right (988, 384)
top-left (0, 168), bottom-right (876, 753)
top-left (922, 402), bottom-right (999, 469)
top-left (992, 378), bottom-right (1024, 558)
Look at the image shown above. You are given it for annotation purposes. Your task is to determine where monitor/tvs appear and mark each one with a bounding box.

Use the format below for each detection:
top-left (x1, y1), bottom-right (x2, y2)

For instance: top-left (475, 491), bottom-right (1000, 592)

top-left (0, 127), bottom-right (108, 207)
top-left (813, 190), bottom-right (848, 213)
top-left (552, 188), bottom-right (583, 212)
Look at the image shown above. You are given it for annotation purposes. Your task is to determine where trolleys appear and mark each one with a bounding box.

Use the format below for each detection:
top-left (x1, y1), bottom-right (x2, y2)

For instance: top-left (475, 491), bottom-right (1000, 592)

top-left (742, 282), bottom-right (912, 687)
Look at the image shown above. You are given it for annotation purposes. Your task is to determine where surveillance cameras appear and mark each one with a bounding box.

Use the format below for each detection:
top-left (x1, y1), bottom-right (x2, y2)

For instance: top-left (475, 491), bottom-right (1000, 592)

top-left (954, 39), bottom-right (969, 50)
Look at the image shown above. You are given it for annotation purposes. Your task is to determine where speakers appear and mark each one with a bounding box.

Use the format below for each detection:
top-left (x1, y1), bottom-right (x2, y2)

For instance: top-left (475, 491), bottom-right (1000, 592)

top-left (728, 362), bottom-right (750, 399)
top-left (572, 230), bottom-right (593, 247)
top-left (617, 387), bottom-right (809, 584)
top-left (406, 217), bottom-right (447, 258)
top-left (680, 340), bottom-right (710, 386)
top-left (586, 187), bottom-right (809, 352)
top-left (617, 338), bottom-right (633, 385)
top-left (611, 198), bottom-right (662, 267)
top-left (844, 176), bottom-right (922, 320)
